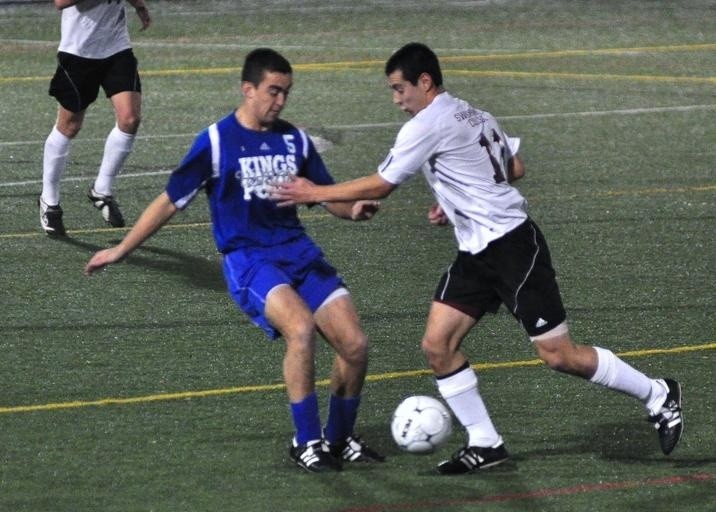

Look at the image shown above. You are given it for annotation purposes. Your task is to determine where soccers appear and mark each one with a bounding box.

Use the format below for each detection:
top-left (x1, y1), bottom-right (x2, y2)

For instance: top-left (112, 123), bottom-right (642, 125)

top-left (390, 395), bottom-right (452, 455)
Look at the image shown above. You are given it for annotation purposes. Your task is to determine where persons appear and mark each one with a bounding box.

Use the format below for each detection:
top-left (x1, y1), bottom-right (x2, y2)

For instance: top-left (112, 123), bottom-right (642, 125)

top-left (37, 0), bottom-right (151, 239)
top-left (265, 41), bottom-right (685, 477)
top-left (83, 46), bottom-right (386, 474)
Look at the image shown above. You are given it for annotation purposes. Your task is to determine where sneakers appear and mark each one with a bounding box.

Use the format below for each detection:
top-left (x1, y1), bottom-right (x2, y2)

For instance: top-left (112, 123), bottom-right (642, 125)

top-left (35, 191), bottom-right (66, 236)
top-left (287, 434), bottom-right (344, 475)
top-left (434, 443), bottom-right (510, 476)
top-left (320, 422), bottom-right (386, 464)
top-left (86, 183), bottom-right (126, 228)
top-left (646, 377), bottom-right (686, 456)
